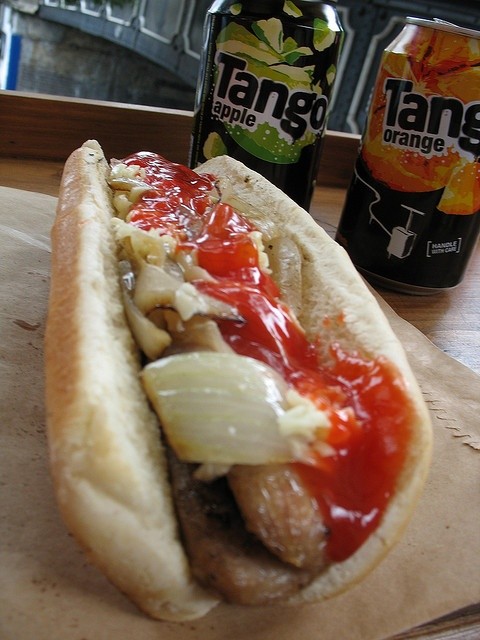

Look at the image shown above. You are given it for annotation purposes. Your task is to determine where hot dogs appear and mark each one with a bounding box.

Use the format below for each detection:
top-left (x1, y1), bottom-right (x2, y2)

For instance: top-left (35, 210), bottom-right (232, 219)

top-left (44, 140), bottom-right (434, 624)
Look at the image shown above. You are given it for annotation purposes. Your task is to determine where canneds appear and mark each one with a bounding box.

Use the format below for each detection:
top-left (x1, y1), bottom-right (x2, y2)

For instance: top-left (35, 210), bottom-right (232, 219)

top-left (331, 17), bottom-right (480, 298)
top-left (187, 0), bottom-right (346, 220)
